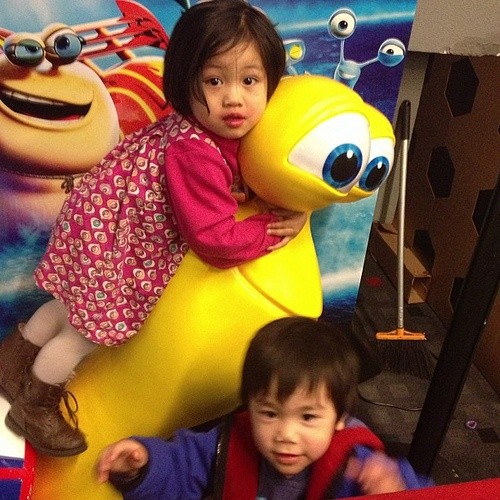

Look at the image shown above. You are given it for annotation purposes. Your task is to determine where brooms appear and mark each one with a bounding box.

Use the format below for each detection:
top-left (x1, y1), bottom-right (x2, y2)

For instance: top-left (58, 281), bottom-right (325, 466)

top-left (375, 100), bottom-right (435, 382)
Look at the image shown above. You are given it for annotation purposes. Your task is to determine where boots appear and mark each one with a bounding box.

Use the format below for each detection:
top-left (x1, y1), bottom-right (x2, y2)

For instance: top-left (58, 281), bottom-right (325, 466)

top-left (6, 367), bottom-right (88, 457)
top-left (1, 322), bottom-right (44, 402)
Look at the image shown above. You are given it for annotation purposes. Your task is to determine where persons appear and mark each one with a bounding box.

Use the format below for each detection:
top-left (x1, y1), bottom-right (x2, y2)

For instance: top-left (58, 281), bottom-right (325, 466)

top-left (1, 1), bottom-right (311, 457)
top-left (94, 317), bottom-right (432, 500)
